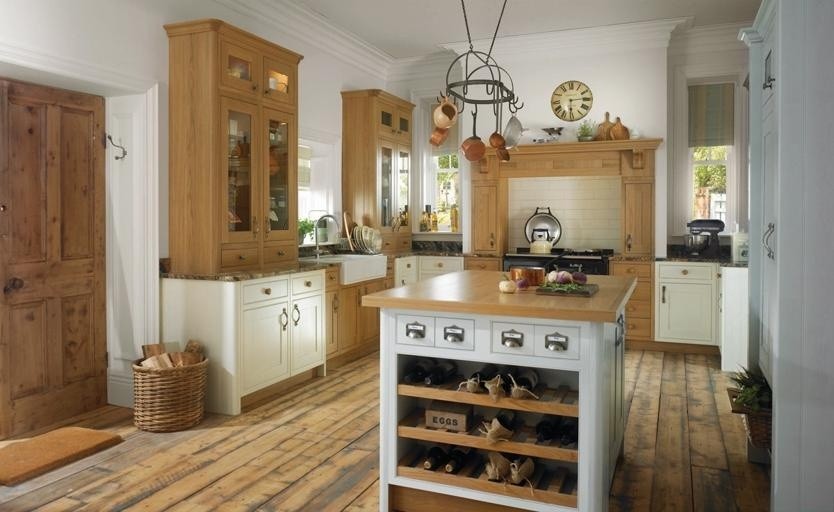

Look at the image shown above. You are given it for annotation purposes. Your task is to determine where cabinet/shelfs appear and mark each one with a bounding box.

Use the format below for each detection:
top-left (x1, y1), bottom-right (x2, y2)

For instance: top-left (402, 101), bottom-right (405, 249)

top-left (716, 267), bottom-right (747, 373)
top-left (354, 267), bottom-right (640, 512)
top-left (619, 175), bottom-right (656, 257)
top-left (737, 0), bottom-right (833, 404)
top-left (610, 257), bottom-right (654, 342)
top-left (160, 263), bottom-right (326, 417)
top-left (167, 94), bottom-right (298, 276)
top-left (471, 178), bottom-right (509, 257)
top-left (341, 133), bottom-right (412, 254)
top-left (326, 256), bottom-right (463, 361)
top-left (465, 256), bottom-right (503, 271)
top-left (161, 18), bottom-right (305, 107)
top-left (339, 85), bottom-right (417, 143)
top-left (655, 261), bottom-right (718, 346)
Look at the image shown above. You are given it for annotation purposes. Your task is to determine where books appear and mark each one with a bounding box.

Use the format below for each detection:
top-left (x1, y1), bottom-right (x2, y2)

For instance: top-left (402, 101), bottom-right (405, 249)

top-left (230, 154), bottom-right (251, 187)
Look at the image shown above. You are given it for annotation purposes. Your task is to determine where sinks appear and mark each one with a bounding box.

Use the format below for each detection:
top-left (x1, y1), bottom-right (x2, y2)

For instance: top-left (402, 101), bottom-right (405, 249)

top-left (299, 254), bottom-right (387, 284)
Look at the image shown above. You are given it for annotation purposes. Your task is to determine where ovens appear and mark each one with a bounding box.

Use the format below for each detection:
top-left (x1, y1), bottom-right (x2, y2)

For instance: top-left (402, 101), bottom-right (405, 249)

top-left (507, 262), bottom-right (605, 275)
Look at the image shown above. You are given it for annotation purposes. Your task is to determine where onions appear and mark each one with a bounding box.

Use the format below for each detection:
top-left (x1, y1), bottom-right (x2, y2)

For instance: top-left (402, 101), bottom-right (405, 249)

top-left (498, 273), bottom-right (518, 294)
top-left (546, 270), bottom-right (588, 285)
top-left (516, 279), bottom-right (530, 291)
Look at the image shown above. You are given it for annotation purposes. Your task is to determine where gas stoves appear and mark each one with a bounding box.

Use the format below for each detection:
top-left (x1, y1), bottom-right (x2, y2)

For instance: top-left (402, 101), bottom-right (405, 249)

top-left (507, 247), bottom-right (611, 256)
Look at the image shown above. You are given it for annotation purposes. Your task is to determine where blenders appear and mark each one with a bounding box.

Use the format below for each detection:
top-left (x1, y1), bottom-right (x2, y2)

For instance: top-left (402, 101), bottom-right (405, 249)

top-left (684, 218), bottom-right (724, 259)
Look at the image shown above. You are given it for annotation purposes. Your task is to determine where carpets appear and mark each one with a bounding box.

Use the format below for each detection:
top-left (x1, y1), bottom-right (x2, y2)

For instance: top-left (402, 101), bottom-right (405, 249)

top-left (0, 424), bottom-right (123, 489)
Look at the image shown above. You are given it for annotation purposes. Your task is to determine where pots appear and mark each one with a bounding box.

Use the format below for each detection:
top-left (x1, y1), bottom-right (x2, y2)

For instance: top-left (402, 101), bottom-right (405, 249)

top-left (458, 113), bottom-right (484, 162)
top-left (428, 94), bottom-right (450, 147)
top-left (501, 115), bottom-right (526, 151)
top-left (525, 204), bottom-right (561, 245)
top-left (510, 250), bottom-right (567, 285)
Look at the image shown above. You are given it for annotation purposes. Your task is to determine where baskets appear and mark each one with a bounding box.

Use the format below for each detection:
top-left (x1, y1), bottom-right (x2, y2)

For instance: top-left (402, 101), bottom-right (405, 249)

top-left (336, 237), bottom-right (382, 254)
top-left (131, 355), bottom-right (209, 433)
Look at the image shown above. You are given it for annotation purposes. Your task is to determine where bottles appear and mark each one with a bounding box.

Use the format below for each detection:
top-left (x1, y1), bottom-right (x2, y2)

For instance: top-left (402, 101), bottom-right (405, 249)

top-left (270, 196), bottom-right (286, 222)
top-left (483, 406), bottom-right (576, 445)
top-left (419, 210), bottom-right (436, 230)
top-left (269, 125), bottom-right (280, 144)
top-left (448, 204), bottom-right (459, 232)
top-left (402, 362), bottom-right (538, 398)
top-left (420, 442), bottom-right (470, 470)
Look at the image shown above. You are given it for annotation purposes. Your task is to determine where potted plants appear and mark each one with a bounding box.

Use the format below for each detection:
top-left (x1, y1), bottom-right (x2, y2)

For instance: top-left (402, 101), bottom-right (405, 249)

top-left (576, 120), bottom-right (595, 143)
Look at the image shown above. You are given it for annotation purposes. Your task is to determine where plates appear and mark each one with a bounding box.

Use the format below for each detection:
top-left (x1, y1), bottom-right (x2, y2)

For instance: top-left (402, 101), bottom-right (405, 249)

top-left (351, 226), bottom-right (380, 254)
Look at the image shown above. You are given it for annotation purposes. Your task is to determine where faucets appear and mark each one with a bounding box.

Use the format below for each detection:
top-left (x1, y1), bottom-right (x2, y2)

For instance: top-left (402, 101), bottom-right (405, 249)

top-left (315, 215), bottom-right (340, 259)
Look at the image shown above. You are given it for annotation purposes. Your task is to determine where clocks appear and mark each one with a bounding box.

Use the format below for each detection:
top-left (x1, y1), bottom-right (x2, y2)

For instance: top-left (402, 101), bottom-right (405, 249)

top-left (549, 79), bottom-right (594, 124)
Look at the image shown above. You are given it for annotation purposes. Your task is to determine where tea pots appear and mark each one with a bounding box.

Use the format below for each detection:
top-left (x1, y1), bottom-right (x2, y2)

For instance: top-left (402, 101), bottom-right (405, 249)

top-left (529, 229), bottom-right (555, 253)
top-left (434, 96), bottom-right (457, 129)
